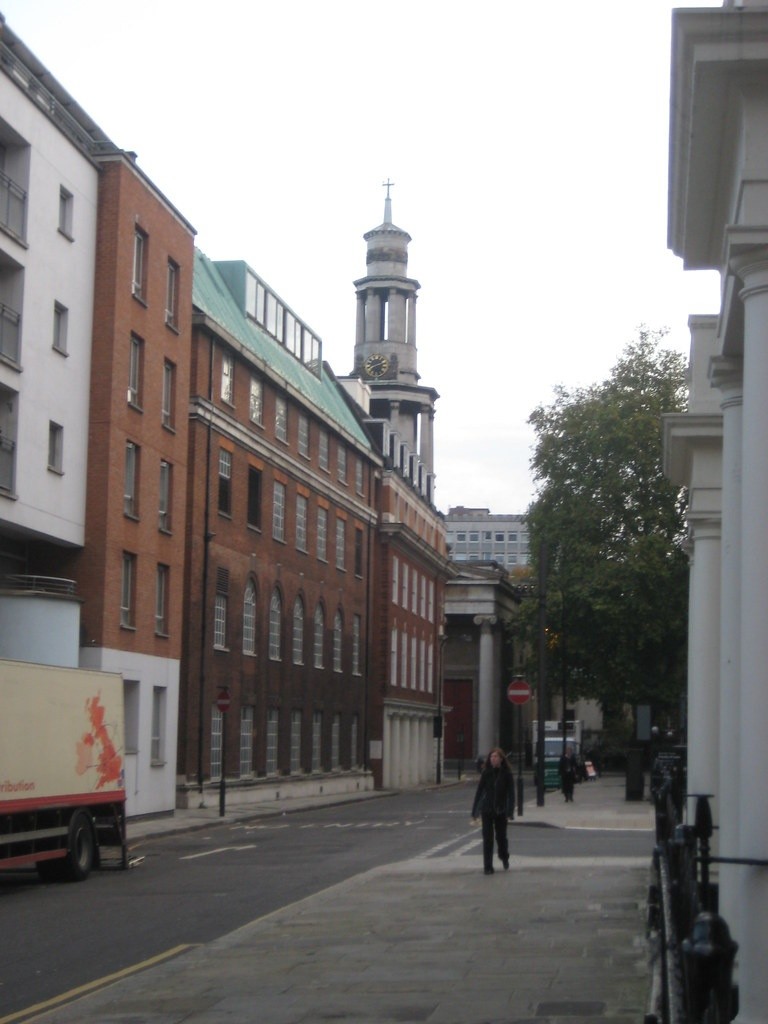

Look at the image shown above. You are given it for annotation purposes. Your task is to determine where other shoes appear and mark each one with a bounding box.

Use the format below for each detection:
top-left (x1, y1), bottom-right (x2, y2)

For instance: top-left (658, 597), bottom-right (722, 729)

top-left (503, 858), bottom-right (510, 869)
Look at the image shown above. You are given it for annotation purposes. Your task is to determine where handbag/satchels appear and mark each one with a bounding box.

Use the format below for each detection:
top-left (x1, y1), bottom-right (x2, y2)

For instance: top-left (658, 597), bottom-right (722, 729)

top-left (474, 799), bottom-right (483, 816)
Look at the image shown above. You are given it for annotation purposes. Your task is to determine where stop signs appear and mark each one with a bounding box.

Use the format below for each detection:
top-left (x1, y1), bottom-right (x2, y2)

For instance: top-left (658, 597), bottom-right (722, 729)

top-left (217, 690), bottom-right (230, 711)
top-left (507, 681), bottom-right (530, 706)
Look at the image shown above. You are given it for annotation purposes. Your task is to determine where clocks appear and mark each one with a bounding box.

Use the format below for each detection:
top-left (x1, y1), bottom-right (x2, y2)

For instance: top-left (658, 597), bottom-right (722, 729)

top-left (364, 353), bottom-right (390, 378)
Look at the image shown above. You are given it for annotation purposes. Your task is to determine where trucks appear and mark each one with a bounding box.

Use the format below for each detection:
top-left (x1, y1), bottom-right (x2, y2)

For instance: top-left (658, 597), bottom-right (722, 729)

top-left (533, 718), bottom-right (583, 784)
top-left (0, 660), bottom-right (145, 885)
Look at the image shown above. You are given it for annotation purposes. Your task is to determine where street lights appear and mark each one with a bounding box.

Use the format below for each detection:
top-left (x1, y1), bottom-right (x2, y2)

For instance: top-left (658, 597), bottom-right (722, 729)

top-left (436, 633), bottom-right (472, 784)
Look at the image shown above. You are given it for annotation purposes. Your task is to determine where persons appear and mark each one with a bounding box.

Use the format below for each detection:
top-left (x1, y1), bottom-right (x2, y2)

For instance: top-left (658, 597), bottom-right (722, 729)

top-left (471, 748), bottom-right (514, 874)
top-left (589, 746), bottom-right (598, 767)
top-left (558, 748), bottom-right (578, 802)
